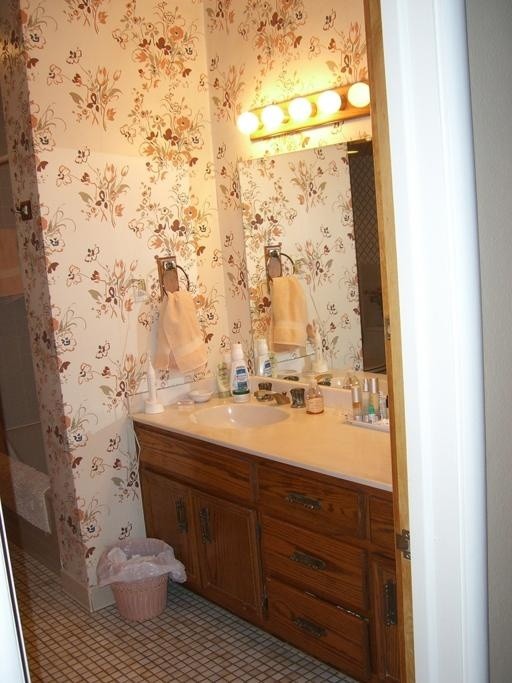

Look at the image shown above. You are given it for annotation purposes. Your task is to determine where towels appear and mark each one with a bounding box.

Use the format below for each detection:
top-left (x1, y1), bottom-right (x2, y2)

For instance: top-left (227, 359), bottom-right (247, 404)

top-left (155, 288), bottom-right (209, 374)
top-left (268, 275), bottom-right (308, 354)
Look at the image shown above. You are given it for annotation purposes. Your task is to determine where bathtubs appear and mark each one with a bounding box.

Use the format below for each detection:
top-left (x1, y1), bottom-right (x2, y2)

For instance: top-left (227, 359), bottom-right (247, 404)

top-left (0, 421), bottom-right (63, 579)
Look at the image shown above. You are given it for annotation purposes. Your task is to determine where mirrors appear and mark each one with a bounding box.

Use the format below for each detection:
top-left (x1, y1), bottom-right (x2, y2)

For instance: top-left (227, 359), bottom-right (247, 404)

top-left (236, 138), bottom-right (400, 397)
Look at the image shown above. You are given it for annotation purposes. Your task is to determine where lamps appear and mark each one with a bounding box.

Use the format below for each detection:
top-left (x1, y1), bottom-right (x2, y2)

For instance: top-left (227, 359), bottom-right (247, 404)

top-left (235, 80), bottom-right (371, 142)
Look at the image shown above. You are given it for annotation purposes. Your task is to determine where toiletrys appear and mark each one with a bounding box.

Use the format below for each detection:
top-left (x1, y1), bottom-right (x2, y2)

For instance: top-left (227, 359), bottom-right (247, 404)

top-left (347, 377), bottom-right (388, 425)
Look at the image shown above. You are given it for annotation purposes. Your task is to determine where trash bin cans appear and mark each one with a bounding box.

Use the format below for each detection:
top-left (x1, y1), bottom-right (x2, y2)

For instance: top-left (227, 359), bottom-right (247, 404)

top-left (103, 537), bottom-right (172, 624)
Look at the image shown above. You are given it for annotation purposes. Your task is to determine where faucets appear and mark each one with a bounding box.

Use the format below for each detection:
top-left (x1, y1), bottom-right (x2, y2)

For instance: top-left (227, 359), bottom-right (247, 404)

top-left (314, 372), bottom-right (333, 387)
top-left (254, 389), bottom-right (291, 404)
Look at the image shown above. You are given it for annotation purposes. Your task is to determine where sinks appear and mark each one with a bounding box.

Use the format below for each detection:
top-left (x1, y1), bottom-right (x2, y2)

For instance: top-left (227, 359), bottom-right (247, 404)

top-left (328, 375), bottom-right (388, 394)
top-left (191, 403), bottom-right (291, 430)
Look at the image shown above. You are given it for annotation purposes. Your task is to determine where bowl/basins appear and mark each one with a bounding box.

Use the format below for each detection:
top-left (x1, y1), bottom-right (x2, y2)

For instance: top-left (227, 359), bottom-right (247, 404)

top-left (188, 389), bottom-right (213, 403)
